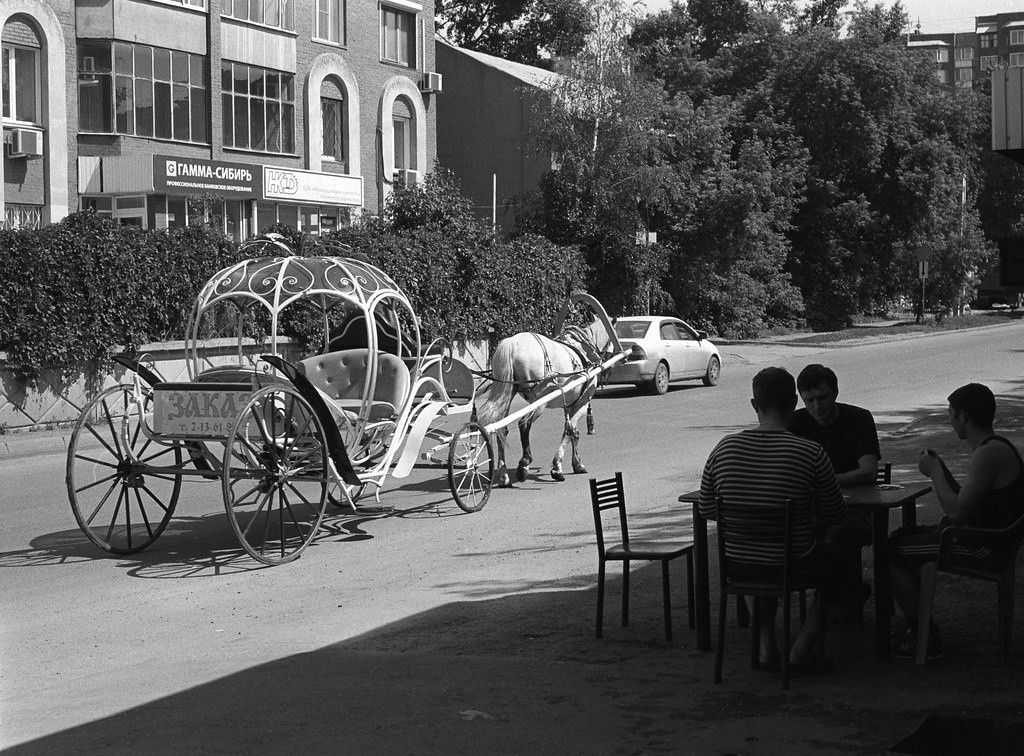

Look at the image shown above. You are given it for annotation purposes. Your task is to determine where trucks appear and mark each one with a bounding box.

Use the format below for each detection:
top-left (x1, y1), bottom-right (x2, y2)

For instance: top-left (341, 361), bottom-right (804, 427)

top-left (970, 237), bottom-right (1024, 309)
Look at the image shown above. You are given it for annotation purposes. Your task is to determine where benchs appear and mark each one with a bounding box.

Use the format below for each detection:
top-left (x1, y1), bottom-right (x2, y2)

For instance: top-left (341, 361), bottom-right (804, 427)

top-left (189, 366), bottom-right (358, 446)
top-left (289, 348), bottom-right (411, 444)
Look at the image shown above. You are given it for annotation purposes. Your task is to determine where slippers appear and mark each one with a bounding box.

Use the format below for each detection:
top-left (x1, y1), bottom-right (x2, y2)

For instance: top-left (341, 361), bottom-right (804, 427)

top-left (760, 656), bottom-right (783, 671)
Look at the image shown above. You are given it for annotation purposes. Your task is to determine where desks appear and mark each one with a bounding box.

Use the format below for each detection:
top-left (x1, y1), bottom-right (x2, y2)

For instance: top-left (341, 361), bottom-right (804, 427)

top-left (678, 482), bottom-right (933, 662)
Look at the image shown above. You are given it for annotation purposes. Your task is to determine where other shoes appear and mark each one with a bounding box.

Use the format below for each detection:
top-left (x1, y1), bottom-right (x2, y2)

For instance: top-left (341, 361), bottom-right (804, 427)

top-left (891, 620), bottom-right (939, 646)
top-left (891, 629), bottom-right (944, 660)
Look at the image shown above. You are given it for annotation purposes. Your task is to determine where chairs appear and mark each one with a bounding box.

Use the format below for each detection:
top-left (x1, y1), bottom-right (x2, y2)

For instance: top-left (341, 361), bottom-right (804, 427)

top-left (713, 495), bottom-right (832, 690)
top-left (915, 514), bottom-right (1024, 667)
top-left (589, 471), bottom-right (694, 642)
top-left (800, 461), bottom-right (892, 631)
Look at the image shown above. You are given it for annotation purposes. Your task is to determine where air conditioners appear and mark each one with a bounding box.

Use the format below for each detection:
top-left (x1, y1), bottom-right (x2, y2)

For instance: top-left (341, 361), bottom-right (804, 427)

top-left (423, 72), bottom-right (443, 93)
top-left (82, 56), bottom-right (96, 81)
top-left (10, 129), bottom-right (43, 157)
top-left (397, 169), bottom-right (420, 191)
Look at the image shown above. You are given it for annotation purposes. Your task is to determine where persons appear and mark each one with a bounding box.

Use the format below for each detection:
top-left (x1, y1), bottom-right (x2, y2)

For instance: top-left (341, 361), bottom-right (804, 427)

top-left (786, 364), bottom-right (882, 555)
top-left (698, 365), bottom-right (852, 679)
top-left (888, 381), bottom-right (1023, 661)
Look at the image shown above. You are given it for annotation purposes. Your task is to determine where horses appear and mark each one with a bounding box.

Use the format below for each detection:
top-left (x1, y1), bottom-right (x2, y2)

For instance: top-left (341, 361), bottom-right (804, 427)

top-left (472, 310), bottom-right (617, 490)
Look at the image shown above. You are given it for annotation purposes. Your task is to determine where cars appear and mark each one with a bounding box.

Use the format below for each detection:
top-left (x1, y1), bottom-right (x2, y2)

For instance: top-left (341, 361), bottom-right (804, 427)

top-left (598, 316), bottom-right (723, 395)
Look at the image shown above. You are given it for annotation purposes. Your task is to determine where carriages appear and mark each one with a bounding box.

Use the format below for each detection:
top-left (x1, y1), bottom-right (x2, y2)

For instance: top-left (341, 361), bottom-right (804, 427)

top-left (65, 255), bottom-right (636, 566)
